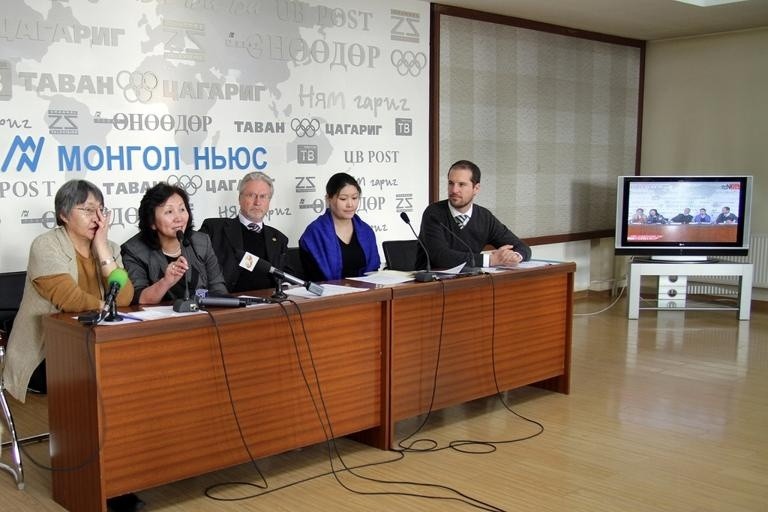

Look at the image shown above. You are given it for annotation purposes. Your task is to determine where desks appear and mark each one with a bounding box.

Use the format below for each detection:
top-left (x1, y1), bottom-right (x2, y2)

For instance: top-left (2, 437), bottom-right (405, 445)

top-left (621, 257), bottom-right (754, 322)
top-left (34, 275), bottom-right (392, 510)
top-left (361, 256), bottom-right (575, 449)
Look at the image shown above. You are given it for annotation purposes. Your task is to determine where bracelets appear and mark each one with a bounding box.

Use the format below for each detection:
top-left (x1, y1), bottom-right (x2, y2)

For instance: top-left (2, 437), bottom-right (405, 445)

top-left (99, 257), bottom-right (115, 267)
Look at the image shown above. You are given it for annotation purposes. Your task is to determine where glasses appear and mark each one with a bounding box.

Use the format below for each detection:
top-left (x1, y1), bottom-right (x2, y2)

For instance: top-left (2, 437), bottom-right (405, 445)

top-left (71, 205), bottom-right (109, 218)
top-left (243, 192), bottom-right (270, 202)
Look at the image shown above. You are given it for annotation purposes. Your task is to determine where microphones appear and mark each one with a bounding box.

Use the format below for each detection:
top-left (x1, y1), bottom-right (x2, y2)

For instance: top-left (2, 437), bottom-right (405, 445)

top-left (173, 231), bottom-right (199, 312)
top-left (431, 214), bottom-right (482, 276)
top-left (400, 212), bottom-right (437, 282)
top-left (238, 251), bottom-right (324, 296)
top-left (102, 268), bottom-right (128, 317)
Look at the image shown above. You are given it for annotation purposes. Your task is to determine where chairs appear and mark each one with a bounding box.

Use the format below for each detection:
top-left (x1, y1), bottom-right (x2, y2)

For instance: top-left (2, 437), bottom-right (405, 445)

top-left (0, 267), bottom-right (48, 488)
top-left (287, 243), bottom-right (304, 282)
top-left (382, 239), bottom-right (421, 269)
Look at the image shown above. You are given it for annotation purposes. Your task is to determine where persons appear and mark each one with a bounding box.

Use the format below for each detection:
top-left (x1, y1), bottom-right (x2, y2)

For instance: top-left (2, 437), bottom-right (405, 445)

top-left (629, 206), bottom-right (738, 225)
top-left (197, 172), bottom-right (289, 295)
top-left (119, 183), bottom-right (230, 307)
top-left (297, 172), bottom-right (381, 283)
top-left (414, 161), bottom-right (531, 270)
top-left (2, 180), bottom-right (146, 512)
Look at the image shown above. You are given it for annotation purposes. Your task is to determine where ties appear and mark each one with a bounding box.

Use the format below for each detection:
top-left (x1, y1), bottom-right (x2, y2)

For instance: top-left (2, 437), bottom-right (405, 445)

top-left (249, 223), bottom-right (261, 233)
top-left (456, 214), bottom-right (467, 230)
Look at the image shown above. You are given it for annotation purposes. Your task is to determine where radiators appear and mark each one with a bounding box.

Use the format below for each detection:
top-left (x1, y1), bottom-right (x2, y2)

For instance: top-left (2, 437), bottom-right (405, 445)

top-left (679, 230), bottom-right (768, 289)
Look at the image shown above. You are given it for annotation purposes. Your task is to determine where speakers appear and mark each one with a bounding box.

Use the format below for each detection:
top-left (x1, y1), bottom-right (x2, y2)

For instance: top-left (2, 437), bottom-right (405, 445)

top-left (658, 276), bottom-right (687, 307)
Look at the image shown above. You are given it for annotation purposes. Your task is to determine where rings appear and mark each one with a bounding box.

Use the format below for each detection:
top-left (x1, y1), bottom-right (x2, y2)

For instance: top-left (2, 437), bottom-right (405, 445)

top-left (172, 265), bottom-right (176, 270)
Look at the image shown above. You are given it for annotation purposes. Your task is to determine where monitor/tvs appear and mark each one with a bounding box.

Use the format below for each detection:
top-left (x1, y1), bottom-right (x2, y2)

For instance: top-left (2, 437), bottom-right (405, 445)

top-left (614, 175), bottom-right (753, 263)
